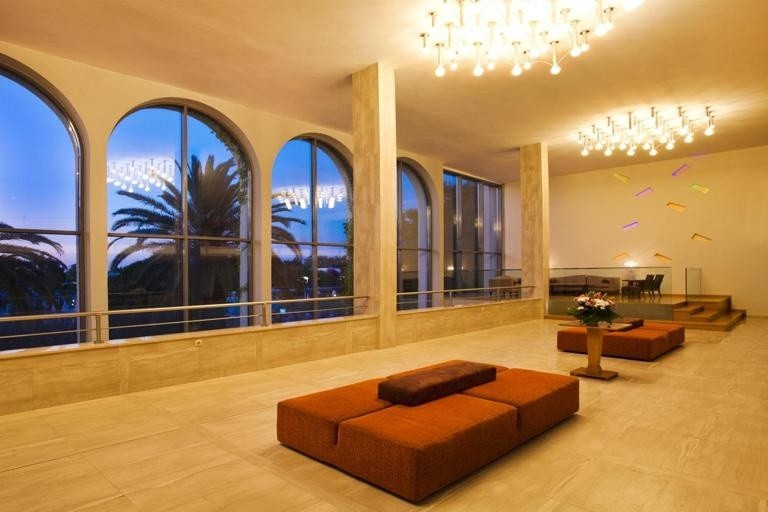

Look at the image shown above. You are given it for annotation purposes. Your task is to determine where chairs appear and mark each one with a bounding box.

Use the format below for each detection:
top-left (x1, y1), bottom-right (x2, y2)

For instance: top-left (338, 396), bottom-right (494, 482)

top-left (487, 274), bottom-right (523, 298)
top-left (550, 274), bottom-right (665, 297)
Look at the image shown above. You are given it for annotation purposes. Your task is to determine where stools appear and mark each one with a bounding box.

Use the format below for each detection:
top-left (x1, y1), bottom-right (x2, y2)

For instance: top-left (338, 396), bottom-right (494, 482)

top-left (276, 377), bottom-right (398, 469)
top-left (337, 392), bottom-right (520, 503)
top-left (557, 326), bottom-right (609, 354)
top-left (461, 369), bottom-right (579, 446)
top-left (638, 323), bottom-right (685, 348)
top-left (603, 327), bottom-right (670, 361)
top-left (386, 359), bottom-right (509, 379)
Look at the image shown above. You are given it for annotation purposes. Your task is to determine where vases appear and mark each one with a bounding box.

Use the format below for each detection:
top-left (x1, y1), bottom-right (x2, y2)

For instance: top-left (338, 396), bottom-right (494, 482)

top-left (570, 327), bottom-right (618, 380)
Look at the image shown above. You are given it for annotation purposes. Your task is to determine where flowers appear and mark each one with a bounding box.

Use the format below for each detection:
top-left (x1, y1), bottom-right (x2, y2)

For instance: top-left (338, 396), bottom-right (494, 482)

top-left (566, 291), bottom-right (623, 328)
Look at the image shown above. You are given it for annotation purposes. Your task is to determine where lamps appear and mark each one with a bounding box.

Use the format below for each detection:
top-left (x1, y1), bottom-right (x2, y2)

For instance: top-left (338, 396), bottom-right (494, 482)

top-left (420, 0), bottom-right (614, 77)
top-left (578, 105), bottom-right (715, 156)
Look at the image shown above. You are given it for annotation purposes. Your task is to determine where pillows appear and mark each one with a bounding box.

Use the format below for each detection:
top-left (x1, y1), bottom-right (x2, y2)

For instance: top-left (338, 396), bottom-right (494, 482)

top-left (377, 360), bottom-right (497, 407)
